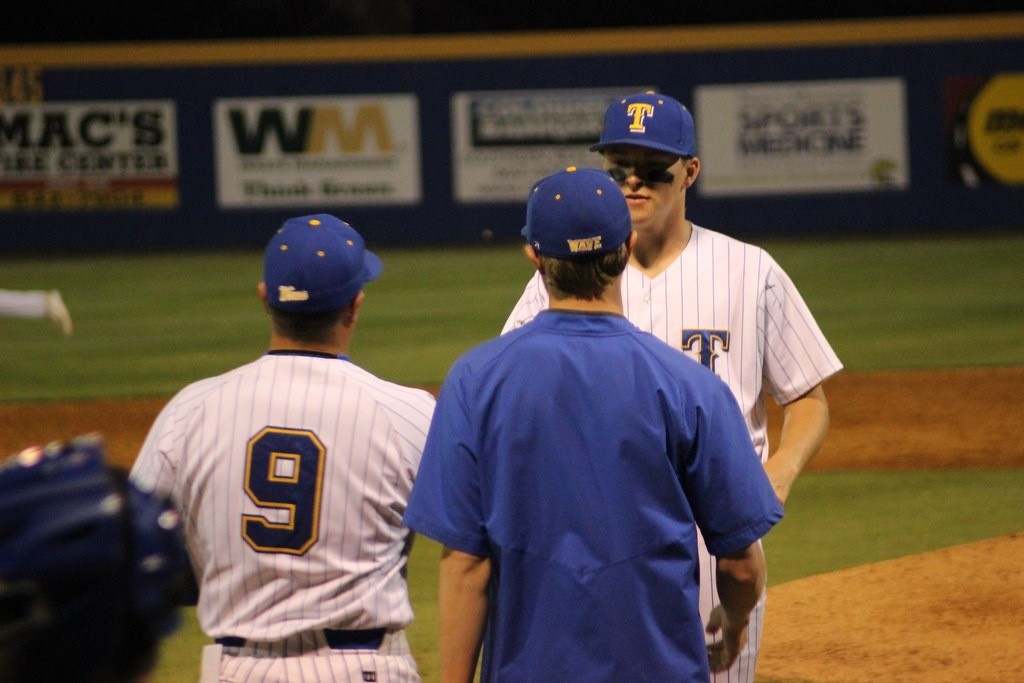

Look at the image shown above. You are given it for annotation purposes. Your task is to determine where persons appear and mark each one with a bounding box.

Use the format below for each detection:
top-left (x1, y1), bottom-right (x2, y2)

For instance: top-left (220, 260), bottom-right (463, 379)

top-left (401, 165), bottom-right (785, 683)
top-left (129, 214), bottom-right (436, 683)
top-left (499, 91), bottom-right (845, 682)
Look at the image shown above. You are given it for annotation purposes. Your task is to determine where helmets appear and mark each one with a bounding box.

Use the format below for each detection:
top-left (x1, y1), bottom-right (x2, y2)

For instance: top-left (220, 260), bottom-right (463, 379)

top-left (0, 433), bottom-right (199, 683)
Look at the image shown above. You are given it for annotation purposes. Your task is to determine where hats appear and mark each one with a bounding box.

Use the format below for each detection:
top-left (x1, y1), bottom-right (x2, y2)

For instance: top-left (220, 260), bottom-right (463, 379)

top-left (262, 213), bottom-right (383, 313)
top-left (589, 90), bottom-right (697, 157)
top-left (521, 166), bottom-right (632, 260)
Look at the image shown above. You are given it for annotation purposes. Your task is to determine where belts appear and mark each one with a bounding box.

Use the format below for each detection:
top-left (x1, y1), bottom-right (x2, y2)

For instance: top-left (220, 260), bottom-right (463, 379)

top-left (214, 629), bottom-right (387, 651)
